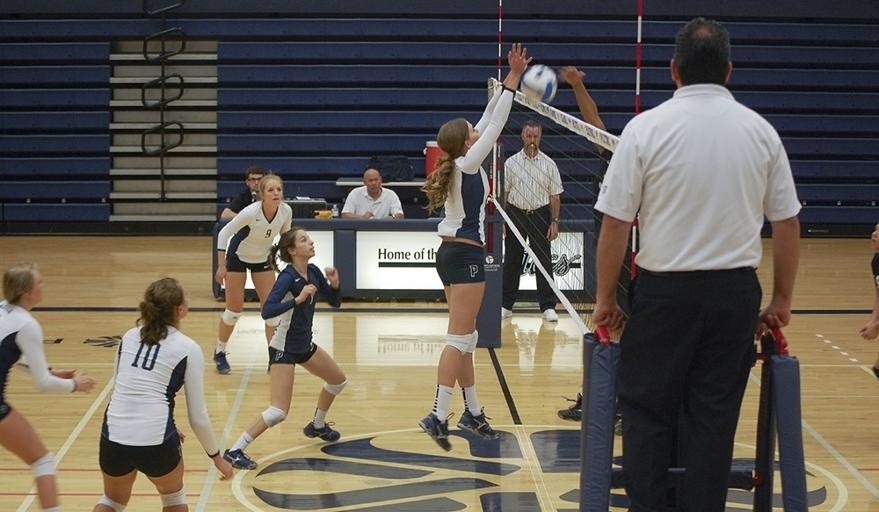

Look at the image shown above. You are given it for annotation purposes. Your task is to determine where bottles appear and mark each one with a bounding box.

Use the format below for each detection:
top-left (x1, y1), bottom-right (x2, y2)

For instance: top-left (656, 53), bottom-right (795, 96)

top-left (331, 205), bottom-right (338, 218)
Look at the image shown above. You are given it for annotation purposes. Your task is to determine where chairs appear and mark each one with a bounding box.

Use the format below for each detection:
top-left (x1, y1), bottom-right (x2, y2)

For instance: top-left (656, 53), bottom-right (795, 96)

top-left (280, 199), bottom-right (327, 219)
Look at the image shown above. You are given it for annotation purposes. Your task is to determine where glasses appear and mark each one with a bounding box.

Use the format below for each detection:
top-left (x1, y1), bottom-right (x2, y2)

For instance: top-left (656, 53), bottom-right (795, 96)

top-left (247, 178), bottom-right (262, 181)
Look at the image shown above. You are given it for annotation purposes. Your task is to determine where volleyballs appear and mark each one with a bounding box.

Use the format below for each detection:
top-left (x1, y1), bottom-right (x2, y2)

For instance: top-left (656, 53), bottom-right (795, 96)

top-left (520, 64), bottom-right (557, 102)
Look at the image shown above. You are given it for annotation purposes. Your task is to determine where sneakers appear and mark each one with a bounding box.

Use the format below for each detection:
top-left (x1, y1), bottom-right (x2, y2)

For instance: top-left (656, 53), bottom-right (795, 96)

top-left (418, 413), bottom-right (452, 452)
top-left (501, 308), bottom-right (512, 319)
top-left (558, 392), bottom-right (583, 420)
top-left (214, 349), bottom-right (231, 374)
top-left (457, 411), bottom-right (501, 440)
top-left (223, 449), bottom-right (258, 469)
top-left (303, 422), bottom-right (340, 441)
top-left (543, 309), bottom-right (559, 321)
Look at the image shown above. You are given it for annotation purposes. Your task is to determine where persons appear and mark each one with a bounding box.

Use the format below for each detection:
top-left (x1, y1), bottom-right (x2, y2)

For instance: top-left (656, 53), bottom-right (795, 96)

top-left (91, 277), bottom-right (234, 512)
top-left (418, 41), bottom-right (534, 453)
top-left (0, 264), bottom-right (98, 512)
top-left (858, 223), bottom-right (879, 377)
top-left (592, 16), bottom-right (803, 512)
top-left (214, 174), bottom-right (293, 374)
top-left (500, 119), bottom-right (565, 322)
top-left (220, 166), bottom-right (265, 219)
top-left (223, 228), bottom-right (348, 470)
top-left (559, 65), bottom-right (625, 435)
top-left (341, 169), bottom-right (404, 220)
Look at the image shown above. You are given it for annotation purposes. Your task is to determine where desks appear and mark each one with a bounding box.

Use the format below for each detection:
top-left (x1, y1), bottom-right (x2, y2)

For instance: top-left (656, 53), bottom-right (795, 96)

top-left (214, 218), bottom-right (598, 301)
top-left (337, 175), bottom-right (429, 208)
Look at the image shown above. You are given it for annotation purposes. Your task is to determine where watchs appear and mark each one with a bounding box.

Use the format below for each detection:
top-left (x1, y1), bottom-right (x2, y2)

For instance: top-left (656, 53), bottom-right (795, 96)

top-left (551, 217), bottom-right (558, 222)
